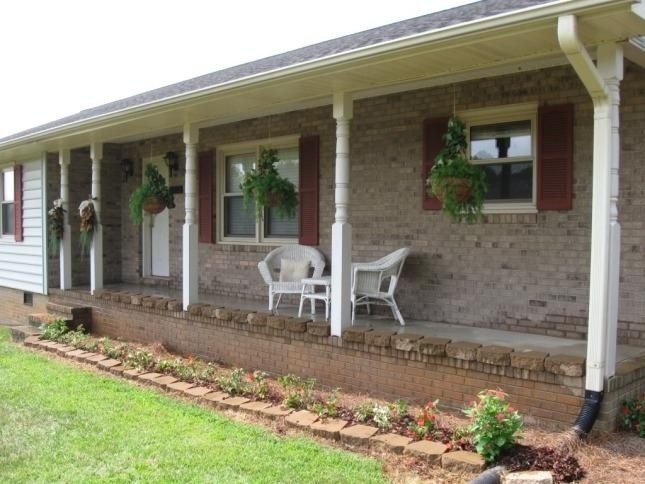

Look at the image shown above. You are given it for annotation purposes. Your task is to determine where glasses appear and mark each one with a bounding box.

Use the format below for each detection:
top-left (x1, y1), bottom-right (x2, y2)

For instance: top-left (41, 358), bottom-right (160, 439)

top-left (121, 157), bottom-right (132, 183)
top-left (162, 151), bottom-right (179, 177)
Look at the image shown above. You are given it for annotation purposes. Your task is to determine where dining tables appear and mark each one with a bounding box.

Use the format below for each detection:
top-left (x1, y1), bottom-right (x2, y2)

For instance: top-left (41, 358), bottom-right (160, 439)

top-left (129, 163), bottom-right (183, 225)
top-left (424, 116), bottom-right (490, 226)
top-left (237, 146), bottom-right (300, 223)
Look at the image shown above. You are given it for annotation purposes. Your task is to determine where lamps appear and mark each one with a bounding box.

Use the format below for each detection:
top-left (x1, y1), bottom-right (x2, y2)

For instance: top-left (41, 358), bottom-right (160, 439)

top-left (256, 244), bottom-right (327, 314)
top-left (351, 246), bottom-right (411, 325)
